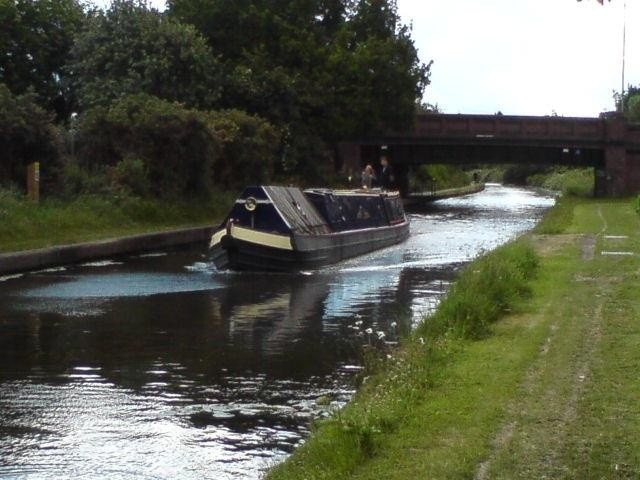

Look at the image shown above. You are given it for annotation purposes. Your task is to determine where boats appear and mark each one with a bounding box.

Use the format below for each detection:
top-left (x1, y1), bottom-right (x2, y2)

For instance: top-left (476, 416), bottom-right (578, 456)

top-left (207, 181), bottom-right (410, 272)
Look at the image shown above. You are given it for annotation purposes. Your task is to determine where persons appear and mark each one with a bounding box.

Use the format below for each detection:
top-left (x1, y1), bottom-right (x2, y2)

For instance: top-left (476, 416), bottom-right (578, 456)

top-left (379, 156), bottom-right (395, 192)
top-left (362, 165), bottom-right (376, 189)
